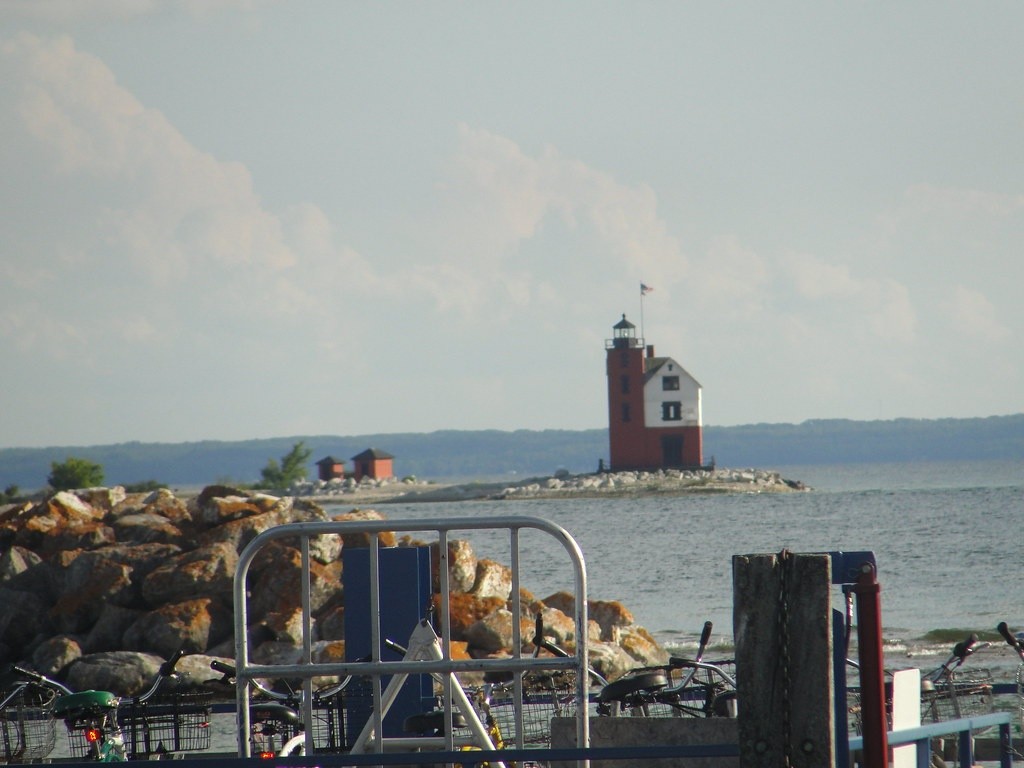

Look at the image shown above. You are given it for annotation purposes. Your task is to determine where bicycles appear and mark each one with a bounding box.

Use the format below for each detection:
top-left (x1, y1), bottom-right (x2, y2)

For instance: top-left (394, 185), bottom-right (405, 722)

top-left (0, 611), bottom-right (738, 768)
top-left (846, 619), bottom-right (1024, 768)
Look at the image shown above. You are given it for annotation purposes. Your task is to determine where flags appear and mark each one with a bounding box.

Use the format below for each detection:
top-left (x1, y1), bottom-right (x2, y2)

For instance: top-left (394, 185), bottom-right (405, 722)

top-left (641, 283), bottom-right (656, 292)
